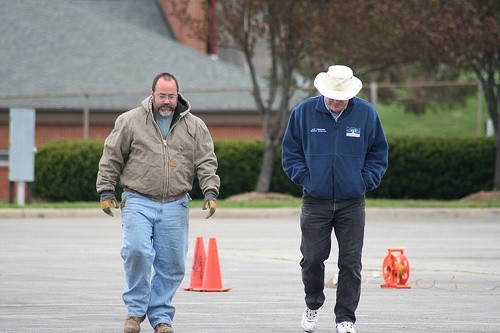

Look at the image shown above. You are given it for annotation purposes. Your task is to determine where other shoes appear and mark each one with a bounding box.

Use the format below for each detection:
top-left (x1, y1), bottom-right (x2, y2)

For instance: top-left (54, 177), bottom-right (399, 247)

top-left (124, 314), bottom-right (146, 333)
top-left (154, 323), bottom-right (175, 333)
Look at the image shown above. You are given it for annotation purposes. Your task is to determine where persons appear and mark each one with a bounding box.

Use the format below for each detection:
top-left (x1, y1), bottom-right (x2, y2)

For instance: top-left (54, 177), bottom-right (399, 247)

top-left (281, 64), bottom-right (389, 333)
top-left (96, 73), bottom-right (221, 333)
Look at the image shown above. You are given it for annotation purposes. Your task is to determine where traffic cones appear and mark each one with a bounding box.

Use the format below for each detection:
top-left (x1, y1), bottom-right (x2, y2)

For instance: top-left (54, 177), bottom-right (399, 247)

top-left (183, 237), bottom-right (207, 291)
top-left (194, 237), bottom-right (232, 293)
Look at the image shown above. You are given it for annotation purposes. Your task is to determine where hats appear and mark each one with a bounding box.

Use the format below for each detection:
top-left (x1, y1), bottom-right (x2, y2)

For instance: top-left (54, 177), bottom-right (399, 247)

top-left (313, 64), bottom-right (363, 100)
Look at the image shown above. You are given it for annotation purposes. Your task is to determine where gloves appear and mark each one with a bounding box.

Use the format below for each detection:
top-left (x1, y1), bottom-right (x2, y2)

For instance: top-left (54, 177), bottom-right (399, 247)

top-left (99, 190), bottom-right (120, 217)
top-left (202, 189), bottom-right (218, 219)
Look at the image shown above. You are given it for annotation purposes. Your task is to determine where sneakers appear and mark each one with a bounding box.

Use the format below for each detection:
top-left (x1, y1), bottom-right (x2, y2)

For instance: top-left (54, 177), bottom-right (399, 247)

top-left (302, 307), bottom-right (319, 333)
top-left (336, 321), bottom-right (356, 333)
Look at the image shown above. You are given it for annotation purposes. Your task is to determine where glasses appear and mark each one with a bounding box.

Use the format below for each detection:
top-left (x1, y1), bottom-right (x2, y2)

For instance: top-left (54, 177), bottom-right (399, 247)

top-left (152, 92), bottom-right (178, 101)
